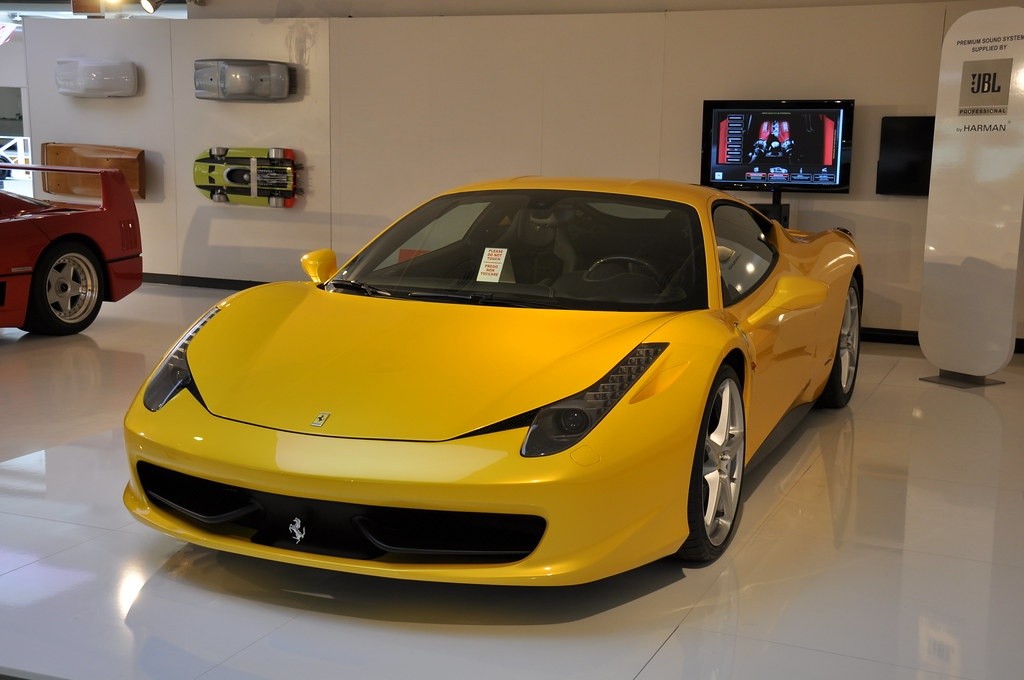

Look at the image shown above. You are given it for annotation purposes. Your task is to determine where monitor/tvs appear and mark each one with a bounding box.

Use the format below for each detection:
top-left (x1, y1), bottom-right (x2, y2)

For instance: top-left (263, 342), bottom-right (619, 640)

top-left (700, 98), bottom-right (855, 193)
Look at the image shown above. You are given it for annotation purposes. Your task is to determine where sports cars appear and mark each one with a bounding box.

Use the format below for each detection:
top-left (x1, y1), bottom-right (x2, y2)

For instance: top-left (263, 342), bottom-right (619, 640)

top-left (0, 162), bottom-right (143, 337)
top-left (124, 176), bottom-right (866, 589)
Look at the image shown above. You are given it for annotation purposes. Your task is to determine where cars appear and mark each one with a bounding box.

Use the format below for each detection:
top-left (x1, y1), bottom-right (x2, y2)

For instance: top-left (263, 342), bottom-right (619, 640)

top-left (193, 145), bottom-right (305, 208)
top-left (55, 58), bottom-right (138, 99)
top-left (194, 58), bottom-right (289, 101)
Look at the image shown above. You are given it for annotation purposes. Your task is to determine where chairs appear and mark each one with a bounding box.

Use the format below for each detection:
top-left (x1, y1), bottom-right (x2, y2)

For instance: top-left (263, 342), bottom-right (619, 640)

top-left (483, 206), bottom-right (576, 287)
